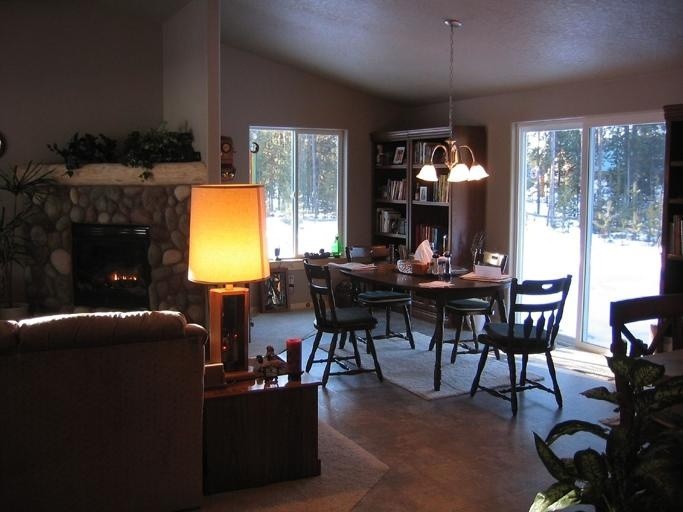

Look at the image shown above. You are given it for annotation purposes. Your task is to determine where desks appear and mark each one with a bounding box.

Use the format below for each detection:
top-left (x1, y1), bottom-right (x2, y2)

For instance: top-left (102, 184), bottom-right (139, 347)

top-left (339, 263), bottom-right (512, 391)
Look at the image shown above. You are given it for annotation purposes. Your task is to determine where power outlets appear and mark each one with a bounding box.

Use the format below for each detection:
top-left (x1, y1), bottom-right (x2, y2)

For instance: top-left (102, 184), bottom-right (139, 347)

top-left (288, 276), bottom-right (294, 294)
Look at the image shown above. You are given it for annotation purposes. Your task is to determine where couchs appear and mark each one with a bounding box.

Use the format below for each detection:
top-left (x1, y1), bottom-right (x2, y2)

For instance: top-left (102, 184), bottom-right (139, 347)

top-left (0, 309), bottom-right (208, 512)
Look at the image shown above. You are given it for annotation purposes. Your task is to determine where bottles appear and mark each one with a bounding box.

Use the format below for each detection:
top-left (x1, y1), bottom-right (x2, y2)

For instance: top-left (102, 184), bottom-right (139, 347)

top-left (333, 236), bottom-right (344, 258)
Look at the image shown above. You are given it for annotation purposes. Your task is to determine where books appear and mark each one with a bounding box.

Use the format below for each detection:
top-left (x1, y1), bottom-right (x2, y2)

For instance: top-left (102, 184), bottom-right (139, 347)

top-left (413, 140), bottom-right (434, 162)
top-left (415, 223), bottom-right (447, 255)
top-left (375, 207), bottom-right (405, 233)
top-left (667, 213), bottom-right (682, 251)
top-left (432, 173), bottom-right (448, 202)
top-left (384, 179), bottom-right (404, 199)
top-left (328, 262), bottom-right (378, 272)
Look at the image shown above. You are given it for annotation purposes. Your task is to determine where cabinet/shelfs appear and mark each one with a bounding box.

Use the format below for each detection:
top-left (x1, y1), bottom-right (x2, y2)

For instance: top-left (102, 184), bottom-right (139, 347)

top-left (204, 357), bottom-right (321, 494)
top-left (654, 104), bottom-right (683, 353)
top-left (370, 124), bottom-right (487, 328)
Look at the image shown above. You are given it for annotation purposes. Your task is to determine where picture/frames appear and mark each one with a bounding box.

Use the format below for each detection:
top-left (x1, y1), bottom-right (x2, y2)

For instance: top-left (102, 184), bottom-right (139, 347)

top-left (392, 147), bottom-right (405, 164)
top-left (260, 267), bottom-right (291, 314)
top-left (419, 186), bottom-right (428, 201)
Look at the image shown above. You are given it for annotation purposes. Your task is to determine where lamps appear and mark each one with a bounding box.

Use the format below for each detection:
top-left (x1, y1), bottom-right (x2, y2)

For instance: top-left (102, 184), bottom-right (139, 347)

top-left (189, 185), bottom-right (271, 383)
top-left (415, 19), bottom-right (490, 182)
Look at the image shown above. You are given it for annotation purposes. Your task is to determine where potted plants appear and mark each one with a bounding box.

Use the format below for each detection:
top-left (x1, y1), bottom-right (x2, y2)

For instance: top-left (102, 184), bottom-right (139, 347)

top-left (0, 161), bottom-right (66, 319)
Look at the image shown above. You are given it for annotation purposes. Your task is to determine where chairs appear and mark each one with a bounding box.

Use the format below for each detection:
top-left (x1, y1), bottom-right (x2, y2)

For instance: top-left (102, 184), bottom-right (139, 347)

top-left (469, 275), bottom-right (572, 418)
top-left (345, 244), bottom-right (416, 353)
top-left (428, 248), bottom-right (508, 365)
top-left (303, 258), bottom-right (385, 387)
top-left (610, 295), bottom-right (683, 433)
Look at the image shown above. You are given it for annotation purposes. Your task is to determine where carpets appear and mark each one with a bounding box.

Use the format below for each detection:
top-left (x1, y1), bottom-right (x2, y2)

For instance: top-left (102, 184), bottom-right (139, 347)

top-left (202, 416), bottom-right (391, 512)
top-left (318, 330), bottom-right (543, 400)
